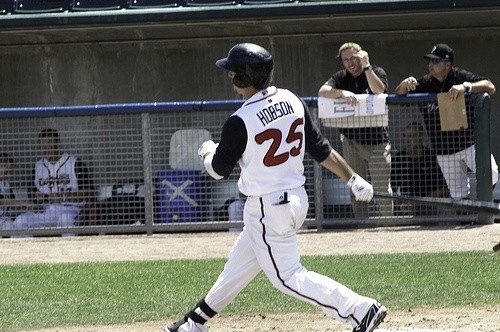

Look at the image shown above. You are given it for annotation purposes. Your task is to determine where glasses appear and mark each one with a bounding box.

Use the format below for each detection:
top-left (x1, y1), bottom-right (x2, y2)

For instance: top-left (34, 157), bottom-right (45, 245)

top-left (428, 57), bottom-right (449, 64)
top-left (342, 57), bottom-right (360, 65)
top-left (402, 133), bottom-right (416, 138)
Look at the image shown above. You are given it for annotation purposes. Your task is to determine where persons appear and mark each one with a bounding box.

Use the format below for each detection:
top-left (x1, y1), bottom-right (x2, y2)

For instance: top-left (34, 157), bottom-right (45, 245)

top-left (14, 128), bottom-right (96, 240)
top-left (396, 44), bottom-right (496, 226)
top-left (0, 152), bottom-right (38, 238)
top-left (165, 43), bottom-right (386, 332)
top-left (391, 122), bottom-right (457, 225)
top-left (318, 42), bottom-right (394, 229)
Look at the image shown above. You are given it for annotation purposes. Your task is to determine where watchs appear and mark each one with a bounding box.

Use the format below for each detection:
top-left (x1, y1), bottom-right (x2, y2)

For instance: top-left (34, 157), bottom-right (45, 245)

top-left (363, 65), bottom-right (372, 72)
top-left (463, 81), bottom-right (471, 93)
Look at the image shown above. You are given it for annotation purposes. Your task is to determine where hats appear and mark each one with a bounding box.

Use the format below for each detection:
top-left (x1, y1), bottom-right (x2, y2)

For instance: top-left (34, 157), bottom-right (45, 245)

top-left (335, 42), bottom-right (361, 61)
top-left (422, 43), bottom-right (455, 62)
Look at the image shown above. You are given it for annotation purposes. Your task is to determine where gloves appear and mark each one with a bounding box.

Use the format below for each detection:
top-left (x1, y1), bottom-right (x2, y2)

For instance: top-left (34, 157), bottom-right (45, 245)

top-left (197, 140), bottom-right (220, 160)
top-left (347, 172), bottom-right (374, 201)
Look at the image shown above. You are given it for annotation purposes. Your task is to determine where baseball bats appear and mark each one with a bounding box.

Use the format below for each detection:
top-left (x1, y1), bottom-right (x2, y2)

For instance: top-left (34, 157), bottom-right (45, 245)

top-left (371, 190), bottom-right (500, 215)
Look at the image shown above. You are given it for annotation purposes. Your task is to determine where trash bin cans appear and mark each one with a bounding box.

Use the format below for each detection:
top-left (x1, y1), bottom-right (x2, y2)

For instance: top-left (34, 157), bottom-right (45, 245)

top-left (160, 169), bottom-right (206, 223)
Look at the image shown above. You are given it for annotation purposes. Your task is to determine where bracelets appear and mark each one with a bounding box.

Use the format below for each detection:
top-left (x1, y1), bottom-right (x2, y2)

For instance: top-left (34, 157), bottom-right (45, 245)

top-left (338, 89), bottom-right (341, 97)
top-left (43, 193), bottom-right (49, 203)
top-left (61, 193), bottom-right (65, 202)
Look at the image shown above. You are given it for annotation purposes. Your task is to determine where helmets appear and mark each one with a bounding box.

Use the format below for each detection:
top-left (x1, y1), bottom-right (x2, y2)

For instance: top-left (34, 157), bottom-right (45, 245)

top-left (215, 42), bottom-right (273, 88)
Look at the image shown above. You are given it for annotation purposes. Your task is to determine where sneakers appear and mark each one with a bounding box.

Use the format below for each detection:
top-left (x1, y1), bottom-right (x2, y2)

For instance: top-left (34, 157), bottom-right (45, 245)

top-left (352, 302), bottom-right (388, 332)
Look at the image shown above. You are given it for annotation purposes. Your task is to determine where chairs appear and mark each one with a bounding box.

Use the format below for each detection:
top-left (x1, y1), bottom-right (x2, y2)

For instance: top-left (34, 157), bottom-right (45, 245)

top-left (0, 0), bottom-right (236, 15)
